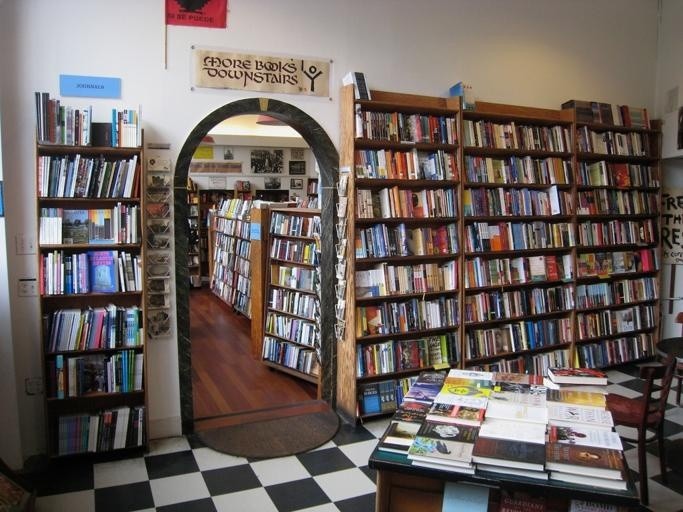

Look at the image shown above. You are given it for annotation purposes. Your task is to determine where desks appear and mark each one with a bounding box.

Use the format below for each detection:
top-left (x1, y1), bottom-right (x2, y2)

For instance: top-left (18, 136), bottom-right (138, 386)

top-left (369, 365), bottom-right (645, 512)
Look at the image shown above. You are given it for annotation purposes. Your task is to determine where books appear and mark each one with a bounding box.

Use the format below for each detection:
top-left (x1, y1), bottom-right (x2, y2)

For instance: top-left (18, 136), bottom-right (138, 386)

top-left (450, 82), bottom-right (475, 112)
top-left (262, 199), bottom-right (321, 375)
top-left (35, 91), bottom-right (142, 458)
top-left (342, 72), bottom-right (372, 100)
top-left (372, 367), bottom-right (628, 490)
top-left (190, 195), bottom-right (275, 318)
top-left (336, 175), bottom-right (348, 338)
top-left (464, 120), bottom-right (574, 374)
top-left (561, 99), bottom-right (662, 369)
top-left (354, 103), bottom-right (458, 414)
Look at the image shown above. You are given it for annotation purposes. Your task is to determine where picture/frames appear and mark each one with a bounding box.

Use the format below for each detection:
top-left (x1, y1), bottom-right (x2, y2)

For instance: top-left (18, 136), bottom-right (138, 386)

top-left (290, 178), bottom-right (304, 189)
top-left (288, 160), bottom-right (306, 175)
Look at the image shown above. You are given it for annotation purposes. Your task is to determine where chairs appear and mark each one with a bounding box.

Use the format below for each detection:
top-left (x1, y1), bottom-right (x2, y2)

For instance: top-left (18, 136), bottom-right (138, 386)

top-left (655, 311), bottom-right (683, 405)
top-left (606, 358), bottom-right (677, 507)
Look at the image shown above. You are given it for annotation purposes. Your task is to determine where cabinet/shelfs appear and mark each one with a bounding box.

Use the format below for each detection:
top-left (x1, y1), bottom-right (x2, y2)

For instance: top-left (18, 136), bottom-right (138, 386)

top-left (335, 78), bottom-right (664, 431)
top-left (34, 125), bottom-right (151, 469)
top-left (185, 187), bottom-right (322, 390)
